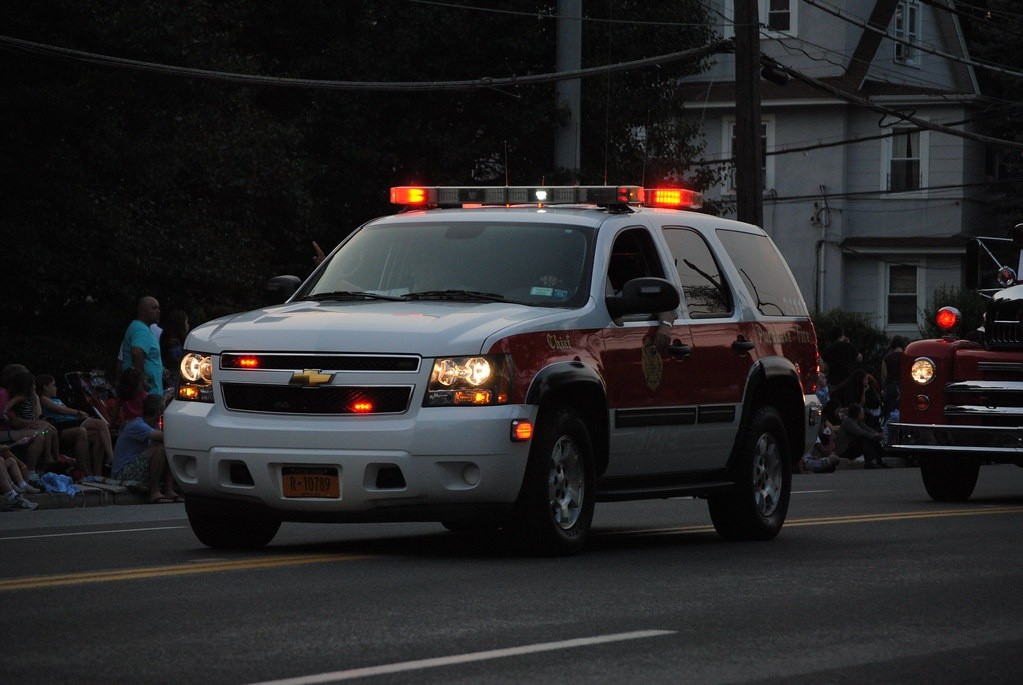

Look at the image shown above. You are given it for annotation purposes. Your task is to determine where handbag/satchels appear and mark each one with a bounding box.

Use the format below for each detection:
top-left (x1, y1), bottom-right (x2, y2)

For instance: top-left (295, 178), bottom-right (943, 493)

top-left (866, 382), bottom-right (882, 410)
top-left (40, 472), bottom-right (81, 499)
top-left (71, 480), bottom-right (128, 508)
top-left (64, 459), bottom-right (95, 482)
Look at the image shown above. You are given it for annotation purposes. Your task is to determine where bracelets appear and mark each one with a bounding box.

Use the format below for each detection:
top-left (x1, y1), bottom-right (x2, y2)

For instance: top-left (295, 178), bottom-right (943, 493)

top-left (77, 409), bottom-right (80, 416)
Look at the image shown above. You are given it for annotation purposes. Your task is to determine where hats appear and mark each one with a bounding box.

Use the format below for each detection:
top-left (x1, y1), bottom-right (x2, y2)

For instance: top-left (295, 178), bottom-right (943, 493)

top-left (847, 402), bottom-right (864, 417)
top-left (1, 364), bottom-right (30, 380)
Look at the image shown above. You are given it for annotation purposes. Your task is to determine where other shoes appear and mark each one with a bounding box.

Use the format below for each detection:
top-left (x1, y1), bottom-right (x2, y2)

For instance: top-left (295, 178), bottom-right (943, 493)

top-left (863, 458), bottom-right (893, 469)
top-left (104, 463), bottom-right (113, 469)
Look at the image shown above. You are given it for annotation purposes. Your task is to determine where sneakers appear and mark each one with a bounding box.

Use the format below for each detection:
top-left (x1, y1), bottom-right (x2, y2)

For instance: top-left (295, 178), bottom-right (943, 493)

top-left (9, 483), bottom-right (26, 494)
top-left (16, 482), bottom-right (41, 494)
top-left (1, 493), bottom-right (38, 512)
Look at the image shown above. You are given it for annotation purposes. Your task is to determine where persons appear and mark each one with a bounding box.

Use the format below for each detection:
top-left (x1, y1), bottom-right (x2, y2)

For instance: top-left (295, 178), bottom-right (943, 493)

top-left (0, 295), bottom-right (188, 511)
top-left (539, 271), bottom-right (613, 298)
top-left (798, 331), bottom-right (912, 473)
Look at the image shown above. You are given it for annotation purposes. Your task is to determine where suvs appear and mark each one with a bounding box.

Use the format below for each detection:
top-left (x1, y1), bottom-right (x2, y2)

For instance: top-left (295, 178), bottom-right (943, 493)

top-left (160, 181), bottom-right (828, 551)
top-left (888, 219), bottom-right (1022, 502)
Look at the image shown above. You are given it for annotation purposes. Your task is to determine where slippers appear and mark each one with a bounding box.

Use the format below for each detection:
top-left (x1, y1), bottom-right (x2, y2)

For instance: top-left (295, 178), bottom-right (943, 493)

top-left (146, 494), bottom-right (174, 504)
top-left (162, 491), bottom-right (186, 502)
